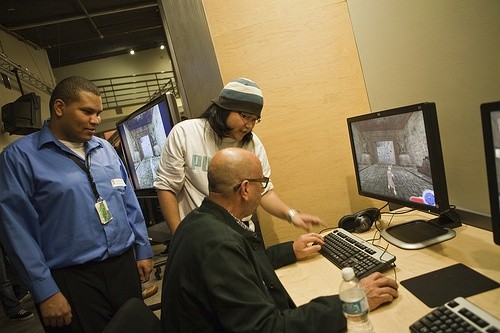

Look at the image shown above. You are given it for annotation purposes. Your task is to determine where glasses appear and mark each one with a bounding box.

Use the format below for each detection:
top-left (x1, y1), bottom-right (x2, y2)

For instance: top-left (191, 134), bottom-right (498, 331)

top-left (234, 175), bottom-right (270, 193)
top-left (236, 110), bottom-right (261, 124)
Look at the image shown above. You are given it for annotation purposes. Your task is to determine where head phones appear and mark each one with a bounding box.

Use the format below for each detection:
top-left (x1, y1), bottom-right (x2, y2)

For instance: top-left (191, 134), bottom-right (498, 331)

top-left (338, 207), bottom-right (381, 233)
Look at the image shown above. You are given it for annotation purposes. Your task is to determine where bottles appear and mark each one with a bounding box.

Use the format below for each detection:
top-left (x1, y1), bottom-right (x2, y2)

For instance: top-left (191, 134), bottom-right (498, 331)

top-left (339, 267), bottom-right (374, 333)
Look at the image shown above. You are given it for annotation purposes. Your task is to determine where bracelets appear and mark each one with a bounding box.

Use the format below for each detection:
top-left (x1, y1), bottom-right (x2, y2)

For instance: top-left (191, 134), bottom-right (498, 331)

top-left (285, 208), bottom-right (300, 224)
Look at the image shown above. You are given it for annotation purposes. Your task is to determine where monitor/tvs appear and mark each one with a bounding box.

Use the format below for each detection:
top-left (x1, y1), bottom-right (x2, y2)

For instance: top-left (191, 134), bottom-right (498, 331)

top-left (480, 101), bottom-right (500, 246)
top-left (347, 102), bottom-right (456, 250)
top-left (116, 92), bottom-right (181, 199)
top-left (1, 92), bottom-right (41, 135)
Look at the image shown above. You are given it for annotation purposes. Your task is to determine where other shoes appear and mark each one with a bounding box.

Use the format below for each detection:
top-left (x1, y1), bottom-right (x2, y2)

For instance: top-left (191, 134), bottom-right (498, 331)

top-left (160, 249), bottom-right (168, 256)
top-left (141, 284), bottom-right (158, 299)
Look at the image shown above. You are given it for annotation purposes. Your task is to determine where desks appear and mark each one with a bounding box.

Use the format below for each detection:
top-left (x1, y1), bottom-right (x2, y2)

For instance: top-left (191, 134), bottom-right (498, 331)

top-left (272, 201), bottom-right (500, 333)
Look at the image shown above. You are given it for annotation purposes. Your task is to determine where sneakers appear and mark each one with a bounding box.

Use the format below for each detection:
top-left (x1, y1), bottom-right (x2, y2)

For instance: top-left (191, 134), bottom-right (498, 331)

top-left (19, 290), bottom-right (31, 303)
top-left (9, 309), bottom-right (34, 321)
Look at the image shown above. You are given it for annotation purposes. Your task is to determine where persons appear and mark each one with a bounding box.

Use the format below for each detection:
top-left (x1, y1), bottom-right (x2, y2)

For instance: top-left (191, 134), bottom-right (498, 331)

top-left (160, 148), bottom-right (399, 333)
top-left (0, 76), bottom-right (160, 333)
top-left (0, 250), bottom-right (34, 322)
top-left (154, 78), bottom-right (324, 230)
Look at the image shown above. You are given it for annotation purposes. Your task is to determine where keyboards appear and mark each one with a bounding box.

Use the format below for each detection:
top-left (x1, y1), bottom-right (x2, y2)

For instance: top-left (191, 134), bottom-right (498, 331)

top-left (312, 227), bottom-right (396, 280)
top-left (409, 296), bottom-right (500, 333)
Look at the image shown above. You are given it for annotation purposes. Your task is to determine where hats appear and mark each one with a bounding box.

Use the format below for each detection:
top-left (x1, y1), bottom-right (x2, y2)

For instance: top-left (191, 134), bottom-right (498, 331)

top-left (211, 78), bottom-right (263, 119)
